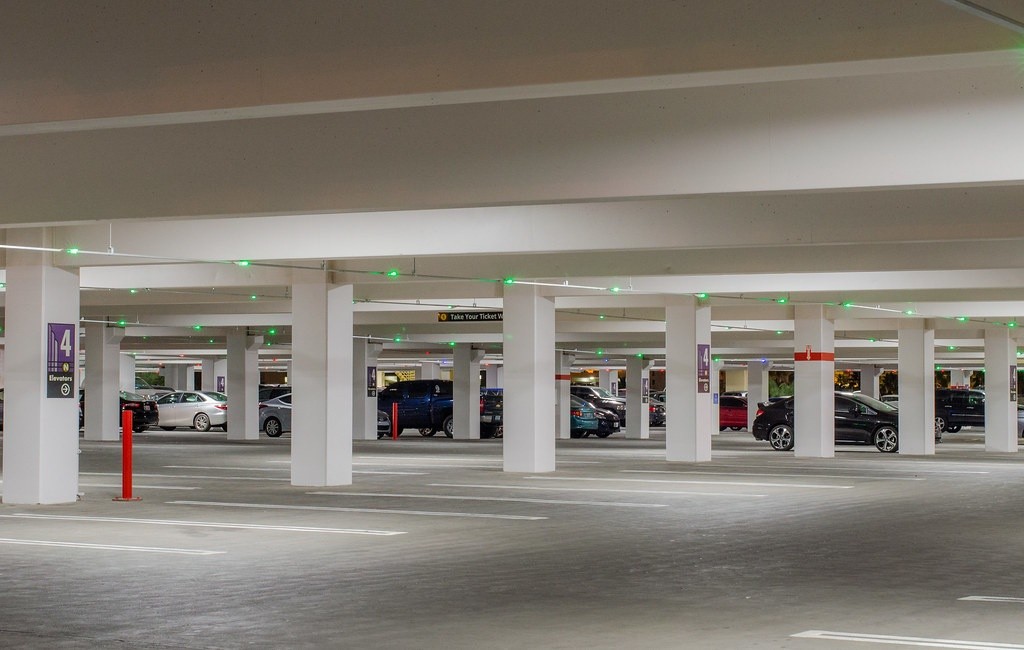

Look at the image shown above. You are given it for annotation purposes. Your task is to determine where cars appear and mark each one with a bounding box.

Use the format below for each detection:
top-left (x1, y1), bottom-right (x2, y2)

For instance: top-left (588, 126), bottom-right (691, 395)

top-left (155, 390), bottom-right (228, 432)
top-left (0, 387), bottom-right (83, 432)
top-left (719, 395), bottom-right (748, 427)
top-left (570, 394), bottom-right (621, 438)
top-left (649, 398), bottom-right (666, 427)
top-left (259, 392), bottom-right (391, 439)
top-left (751, 391), bottom-right (943, 453)
top-left (78, 389), bottom-right (160, 433)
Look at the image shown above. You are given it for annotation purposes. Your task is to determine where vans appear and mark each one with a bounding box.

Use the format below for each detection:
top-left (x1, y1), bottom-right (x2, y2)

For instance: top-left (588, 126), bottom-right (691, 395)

top-left (135, 377), bottom-right (160, 403)
top-left (152, 384), bottom-right (181, 394)
top-left (570, 386), bottom-right (626, 420)
top-left (935, 387), bottom-right (1024, 432)
top-left (259, 384), bottom-right (292, 403)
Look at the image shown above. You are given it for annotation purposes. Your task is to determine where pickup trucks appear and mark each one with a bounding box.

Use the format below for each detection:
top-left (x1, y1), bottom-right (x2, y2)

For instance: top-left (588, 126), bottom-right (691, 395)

top-left (377, 378), bottom-right (503, 438)
